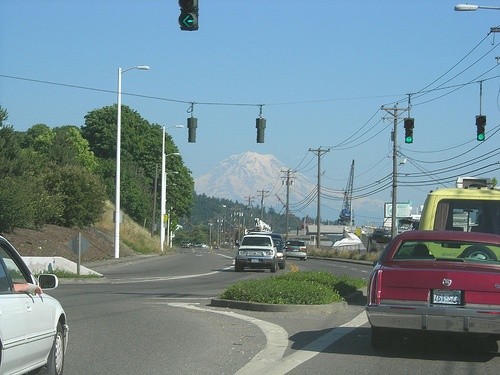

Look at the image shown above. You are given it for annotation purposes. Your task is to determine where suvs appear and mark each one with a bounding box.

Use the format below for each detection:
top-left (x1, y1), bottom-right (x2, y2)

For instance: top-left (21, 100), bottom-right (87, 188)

top-left (415, 186), bottom-right (500, 263)
top-left (234, 232), bottom-right (287, 272)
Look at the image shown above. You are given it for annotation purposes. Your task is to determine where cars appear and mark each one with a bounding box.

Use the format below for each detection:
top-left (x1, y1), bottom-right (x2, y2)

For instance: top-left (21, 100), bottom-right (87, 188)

top-left (365, 230), bottom-right (500, 348)
top-left (283, 239), bottom-right (307, 260)
top-left (0, 235), bottom-right (70, 375)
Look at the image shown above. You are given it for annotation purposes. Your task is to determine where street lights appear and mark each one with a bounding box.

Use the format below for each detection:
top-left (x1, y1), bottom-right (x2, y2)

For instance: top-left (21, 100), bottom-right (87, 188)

top-left (114, 65), bottom-right (150, 259)
top-left (160, 124), bottom-right (184, 251)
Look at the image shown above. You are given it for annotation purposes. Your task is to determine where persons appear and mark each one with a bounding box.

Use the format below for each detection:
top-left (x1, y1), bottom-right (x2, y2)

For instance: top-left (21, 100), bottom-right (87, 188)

top-left (14, 284), bottom-right (44, 300)
top-left (413, 245), bottom-right (429, 259)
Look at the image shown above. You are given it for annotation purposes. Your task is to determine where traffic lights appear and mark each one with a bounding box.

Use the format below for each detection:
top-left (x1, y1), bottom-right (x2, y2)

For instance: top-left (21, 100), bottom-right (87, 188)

top-left (476, 115), bottom-right (486, 141)
top-left (404, 118), bottom-right (414, 143)
top-left (179, 0), bottom-right (199, 30)
